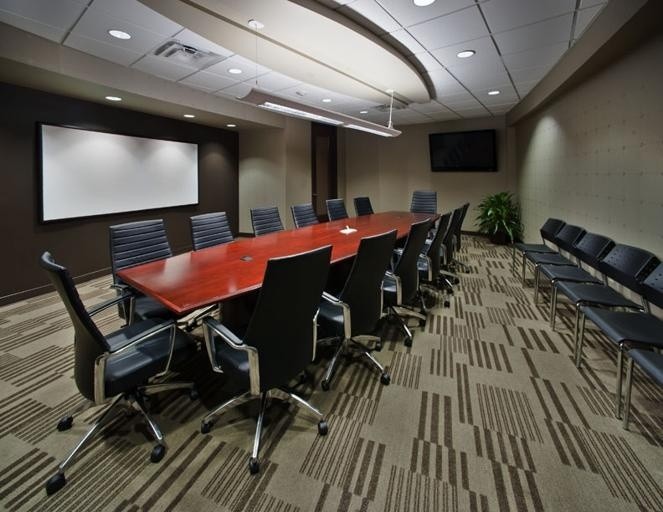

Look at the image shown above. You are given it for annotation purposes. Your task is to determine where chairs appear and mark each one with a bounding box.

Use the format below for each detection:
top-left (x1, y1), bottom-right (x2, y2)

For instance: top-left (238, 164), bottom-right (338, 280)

top-left (325, 198), bottom-right (348, 221)
top-left (510, 215), bottom-right (662, 435)
top-left (41, 252), bottom-right (195, 497)
top-left (317, 229), bottom-right (398, 392)
top-left (291, 202), bottom-right (318, 229)
top-left (110, 218), bottom-right (194, 325)
top-left (200, 243), bottom-right (335, 475)
top-left (250, 205), bottom-right (284, 237)
top-left (372, 190), bottom-right (477, 347)
top-left (190, 211), bottom-right (234, 252)
top-left (353, 196), bottom-right (374, 216)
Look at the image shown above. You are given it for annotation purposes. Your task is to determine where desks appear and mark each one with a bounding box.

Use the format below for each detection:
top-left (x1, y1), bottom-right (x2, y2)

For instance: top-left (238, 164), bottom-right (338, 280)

top-left (117, 210), bottom-right (440, 407)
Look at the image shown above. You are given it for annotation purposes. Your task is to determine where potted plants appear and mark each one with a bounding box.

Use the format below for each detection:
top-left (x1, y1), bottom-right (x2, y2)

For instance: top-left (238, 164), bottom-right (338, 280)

top-left (474, 190), bottom-right (523, 245)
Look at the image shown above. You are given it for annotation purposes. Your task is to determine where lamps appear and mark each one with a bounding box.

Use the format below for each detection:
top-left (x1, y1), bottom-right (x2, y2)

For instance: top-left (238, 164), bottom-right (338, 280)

top-left (236, 16), bottom-right (403, 140)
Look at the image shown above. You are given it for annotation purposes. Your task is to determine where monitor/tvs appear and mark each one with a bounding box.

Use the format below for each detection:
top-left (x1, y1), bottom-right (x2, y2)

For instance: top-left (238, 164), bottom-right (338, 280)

top-left (429, 129), bottom-right (495, 172)
